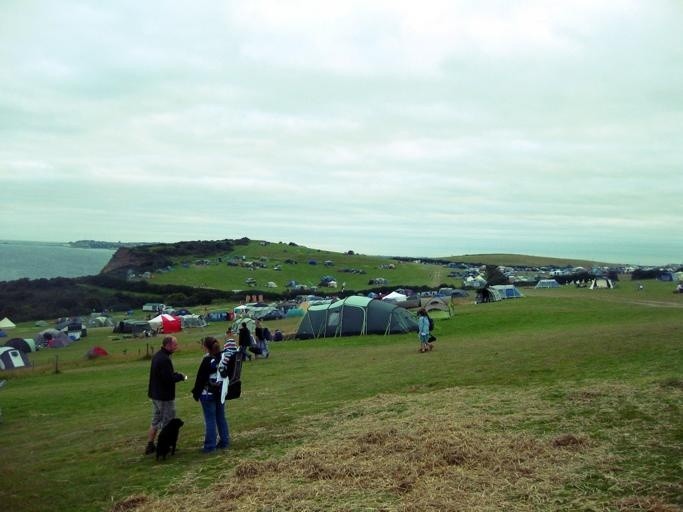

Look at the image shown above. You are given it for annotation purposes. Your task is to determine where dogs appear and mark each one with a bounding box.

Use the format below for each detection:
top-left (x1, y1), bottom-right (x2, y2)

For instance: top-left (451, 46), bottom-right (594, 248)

top-left (155, 417), bottom-right (185, 461)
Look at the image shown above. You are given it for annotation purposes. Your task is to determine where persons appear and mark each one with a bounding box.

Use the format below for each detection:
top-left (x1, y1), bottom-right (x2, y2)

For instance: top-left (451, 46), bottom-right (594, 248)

top-left (190, 336), bottom-right (231, 453)
top-left (143, 335), bottom-right (187, 454)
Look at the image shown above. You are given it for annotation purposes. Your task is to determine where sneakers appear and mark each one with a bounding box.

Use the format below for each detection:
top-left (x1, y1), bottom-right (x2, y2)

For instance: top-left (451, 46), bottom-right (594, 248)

top-left (145, 444), bottom-right (156, 454)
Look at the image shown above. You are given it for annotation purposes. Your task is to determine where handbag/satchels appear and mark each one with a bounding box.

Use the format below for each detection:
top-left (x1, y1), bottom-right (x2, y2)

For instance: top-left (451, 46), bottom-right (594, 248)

top-left (429, 319), bottom-right (433, 331)
top-left (428, 335), bottom-right (436, 342)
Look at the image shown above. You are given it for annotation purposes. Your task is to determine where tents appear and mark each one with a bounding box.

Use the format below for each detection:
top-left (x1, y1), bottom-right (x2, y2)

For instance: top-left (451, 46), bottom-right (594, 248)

top-left (1, 241), bottom-right (683, 372)
